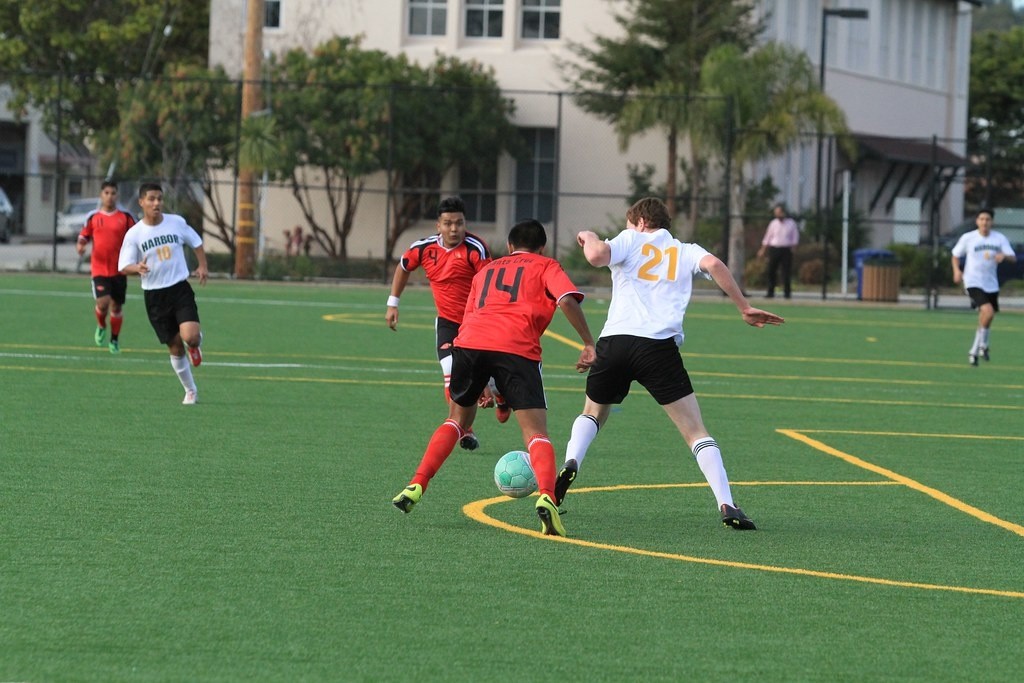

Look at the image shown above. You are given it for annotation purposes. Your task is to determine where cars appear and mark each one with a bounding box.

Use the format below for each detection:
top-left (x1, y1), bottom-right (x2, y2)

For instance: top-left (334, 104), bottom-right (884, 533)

top-left (55, 198), bottom-right (101, 244)
top-left (0, 186), bottom-right (16, 244)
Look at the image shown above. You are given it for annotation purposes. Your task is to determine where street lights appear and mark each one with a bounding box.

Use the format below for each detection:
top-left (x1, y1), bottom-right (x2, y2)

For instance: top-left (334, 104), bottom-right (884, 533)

top-left (815, 7), bottom-right (869, 242)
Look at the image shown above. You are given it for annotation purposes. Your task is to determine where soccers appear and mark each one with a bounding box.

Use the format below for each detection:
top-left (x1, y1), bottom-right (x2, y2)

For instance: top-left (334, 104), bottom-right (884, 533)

top-left (494, 450), bottom-right (538, 498)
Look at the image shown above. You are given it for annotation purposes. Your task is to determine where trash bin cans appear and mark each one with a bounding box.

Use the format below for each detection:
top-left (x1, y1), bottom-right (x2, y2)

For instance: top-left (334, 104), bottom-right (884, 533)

top-left (862, 259), bottom-right (899, 302)
top-left (854, 249), bottom-right (894, 299)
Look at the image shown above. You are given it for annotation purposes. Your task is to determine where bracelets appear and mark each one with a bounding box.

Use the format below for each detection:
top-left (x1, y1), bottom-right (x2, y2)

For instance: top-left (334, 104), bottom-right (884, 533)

top-left (386, 295), bottom-right (400, 307)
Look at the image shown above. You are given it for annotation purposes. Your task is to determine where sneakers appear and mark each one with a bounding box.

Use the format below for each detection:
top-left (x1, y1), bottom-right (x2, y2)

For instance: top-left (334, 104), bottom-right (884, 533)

top-left (720, 503), bottom-right (757, 530)
top-left (392, 484), bottom-right (422, 514)
top-left (535, 494), bottom-right (567, 538)
top-left (977, 347), bottom-right (990, 361)
top-left (968, 351), bottom-right (978, 367)
top-left (554, 459), bottom-right (577, 506)
top-left (495, 397), bottom-right (511, 424)
top-left (459, 432), bottom-right (479, 451)
top-left (186, 346), bottom-right (203, 367)
top-left (109, 340), bottom-right (120, 354)
top-left (183, 389), bottom-right (197, 404)
top-left (94, 327), bottom-right (107, 346)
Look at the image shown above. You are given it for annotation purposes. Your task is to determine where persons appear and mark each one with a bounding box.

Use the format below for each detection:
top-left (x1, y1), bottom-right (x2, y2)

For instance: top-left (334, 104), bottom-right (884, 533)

top-left (118, 183), bottom-right (209, 404)
top-left (757, 203), bottom-right (800, 299)
top-left (384, 196), bottom-right (511, 451)
top-left (478, 384), bottom-right (494, 408)
top-left (950, 209), bottom-right (1017, 366)
top-left (554, 197), bottom-right (785, 530)
top-left (76, 182), bottom-right (136, 355)
top-left (392, 219), bottom-right (597, 538)
